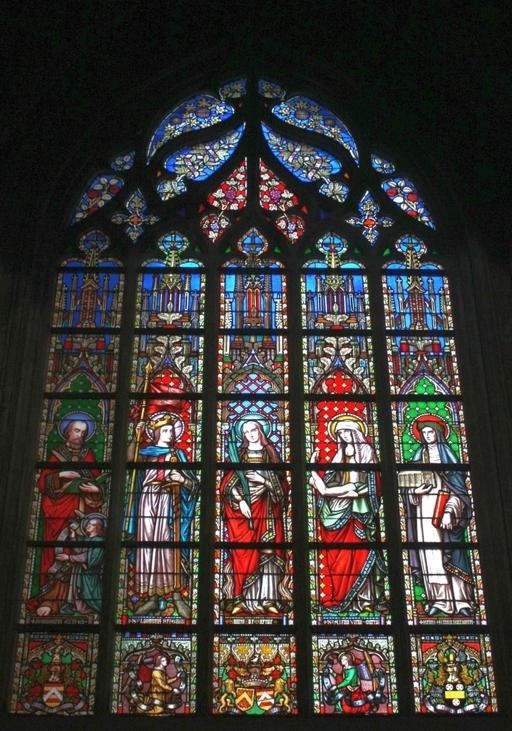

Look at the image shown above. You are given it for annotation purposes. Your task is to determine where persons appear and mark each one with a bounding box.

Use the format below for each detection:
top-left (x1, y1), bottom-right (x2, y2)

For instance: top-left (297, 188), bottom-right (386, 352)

top-left (126, 406), bottom-right (194, 620)
top-left (328, 651), bottom-right (367, 708)
top-left (309, 414), bottom-right (382, 617)
top-left (133, 652), bottom-right (175, 717)
top-left (407, 415), bottom-right (481, 619)
top-left (30, 410), bottom-right (101, 619)
top-left (219, 405), bottom-right (288, 617)
top-left (55, 513), bottom-right (107, 616)
